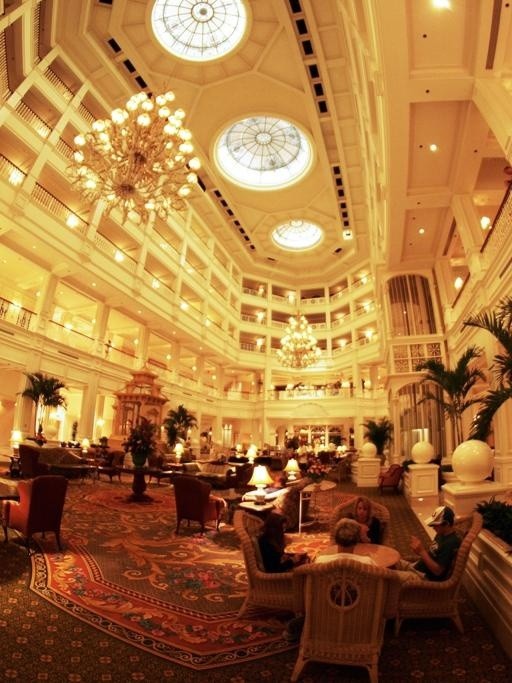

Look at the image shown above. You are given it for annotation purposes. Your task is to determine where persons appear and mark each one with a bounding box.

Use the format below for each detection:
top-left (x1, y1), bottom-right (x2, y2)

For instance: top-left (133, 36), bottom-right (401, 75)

top-left (342, 496), bottom-right (383, 545)
top-left (257, 511), bottom-right (307, 572)
top-left (386, 505), bottom-right (464, 628)
top-left (313, 517), bottom-right (378, 566)
top-left (296, 439), bottom-right (307, 473)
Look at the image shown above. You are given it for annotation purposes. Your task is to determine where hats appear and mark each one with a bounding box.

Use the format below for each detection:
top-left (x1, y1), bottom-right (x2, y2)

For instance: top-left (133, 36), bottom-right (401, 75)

top-left (426, 506), bottom-right (456, 527)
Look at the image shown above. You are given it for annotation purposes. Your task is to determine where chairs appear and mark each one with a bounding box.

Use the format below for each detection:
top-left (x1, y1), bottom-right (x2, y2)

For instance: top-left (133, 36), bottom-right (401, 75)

top-left (97, 450), bottom-right (125, 484)
top-left (330, 494), bottom-right (391, 549)
top-left (2, 474), bottom-right (68, 550)
top-left (378, 464), bottom-right (404, 494)
top-left (233, 508), bottom-right (316, 621)
top-left (292, 559), bottom-right (402, 682)
top-left (394, 510), bottom-right (484, 635)
top-left (171, 475), bottom-right (226, 538)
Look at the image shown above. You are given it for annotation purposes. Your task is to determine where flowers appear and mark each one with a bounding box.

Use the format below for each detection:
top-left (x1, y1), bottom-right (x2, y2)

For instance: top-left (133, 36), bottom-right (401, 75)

top-left (307, 463), bottom-right (333, 475)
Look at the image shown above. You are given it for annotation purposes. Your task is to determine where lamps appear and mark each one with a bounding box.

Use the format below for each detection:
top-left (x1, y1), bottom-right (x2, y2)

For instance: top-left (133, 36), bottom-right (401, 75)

top-left (173, 443), bottom-right (184, 464)
top-left (144, 0), bottom-right (257, 63)
top-left (68, 56), bottom-right (200, 227)
top-left (275, 301), bottom-right (323, 372)
top-left (212, 114), bottom-right (316, 189)
top-left (247, 464), bottom-right (273, 504)
top-left (284, 458), bottom-right (302, 481)
top-left (271, 217), bottom-right (326, 252)
top-left (245, 444), bottom-right (259, 464)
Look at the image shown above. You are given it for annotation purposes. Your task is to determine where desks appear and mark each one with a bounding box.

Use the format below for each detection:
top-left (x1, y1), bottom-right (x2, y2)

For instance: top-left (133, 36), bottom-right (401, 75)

top-left (286, 479), bottom-right (336, 493)
top-left (277, 528), bottom-right (403, 569)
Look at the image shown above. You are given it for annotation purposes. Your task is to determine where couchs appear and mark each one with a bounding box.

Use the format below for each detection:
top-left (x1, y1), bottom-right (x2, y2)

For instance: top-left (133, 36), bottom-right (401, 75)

top-left (19, 443), bottom-right (92, 481)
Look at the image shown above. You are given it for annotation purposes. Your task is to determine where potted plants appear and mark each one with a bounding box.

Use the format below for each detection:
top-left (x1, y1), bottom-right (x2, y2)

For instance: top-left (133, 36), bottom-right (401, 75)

top-left (362, 417), bottom-right (394, 466)
top-left (168, 404), bottom-right (198, 441)
top-left (122, 413), bottom-right (162, 466)
top-left (164, 417), bottom-right (178, 453)
top-left (21, 367), bottom-right (73, 447)
top-left (477, 496), bottom-right (512, 551)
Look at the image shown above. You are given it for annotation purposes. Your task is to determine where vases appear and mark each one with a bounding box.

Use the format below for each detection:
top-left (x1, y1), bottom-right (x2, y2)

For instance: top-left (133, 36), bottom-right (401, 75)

top-left (315, 475), bottom-right (322, 484)
